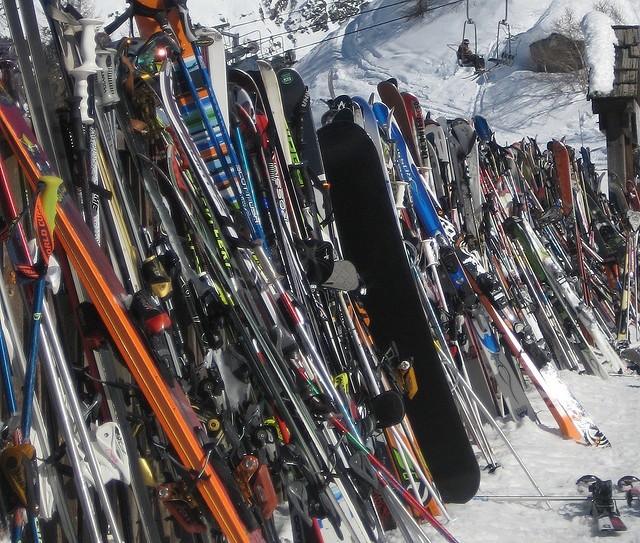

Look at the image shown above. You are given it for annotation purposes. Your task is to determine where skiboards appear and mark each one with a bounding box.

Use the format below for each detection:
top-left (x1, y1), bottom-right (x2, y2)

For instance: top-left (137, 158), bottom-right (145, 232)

top-left (0, 2), bottom-right (333, 543)
top-left (316, 78), bottom-right (639, 503)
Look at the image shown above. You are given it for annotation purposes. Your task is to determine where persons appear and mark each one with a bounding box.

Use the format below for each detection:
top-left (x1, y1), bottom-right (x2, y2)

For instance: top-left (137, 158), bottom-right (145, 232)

top-left (458, 39), bottom-right (484, 74)
